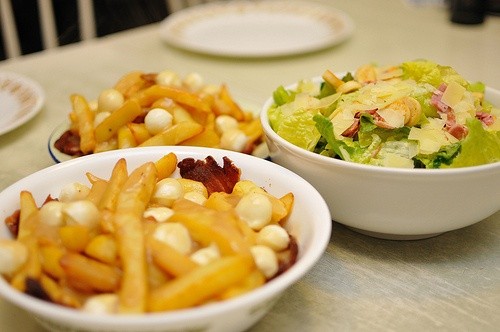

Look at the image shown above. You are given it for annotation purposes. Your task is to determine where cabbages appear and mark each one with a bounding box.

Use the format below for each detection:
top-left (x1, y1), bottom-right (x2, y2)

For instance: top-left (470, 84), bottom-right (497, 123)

top-left (265, 56), bottom-right (500, 171)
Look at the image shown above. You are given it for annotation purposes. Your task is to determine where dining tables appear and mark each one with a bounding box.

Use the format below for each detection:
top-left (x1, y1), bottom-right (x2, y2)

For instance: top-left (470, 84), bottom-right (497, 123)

top-left (1, 0), bottom-right (500, 332)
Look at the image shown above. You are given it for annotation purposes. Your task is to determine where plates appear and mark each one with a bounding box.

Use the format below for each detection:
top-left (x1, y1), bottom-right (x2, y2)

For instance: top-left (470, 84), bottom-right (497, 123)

top-left (160, 0), bottom-right (354, 57)
top-left (0, 72), bottom-right (43, 136)
top-left (49, 105), bottom-right (268, 164)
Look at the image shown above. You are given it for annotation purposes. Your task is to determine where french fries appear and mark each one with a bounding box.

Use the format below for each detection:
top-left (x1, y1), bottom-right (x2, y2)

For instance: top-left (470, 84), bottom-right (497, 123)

top-left (1, 152), bottom-right (295, 316)
top-left (54, 68), bottom-right (264, 155)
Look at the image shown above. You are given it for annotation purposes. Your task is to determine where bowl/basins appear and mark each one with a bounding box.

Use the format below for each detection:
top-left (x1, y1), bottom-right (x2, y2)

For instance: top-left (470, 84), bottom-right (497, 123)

top-left (261, 74), bottom-right (500, 239)
top-left (1, 145), bottom-right (331, 331)
